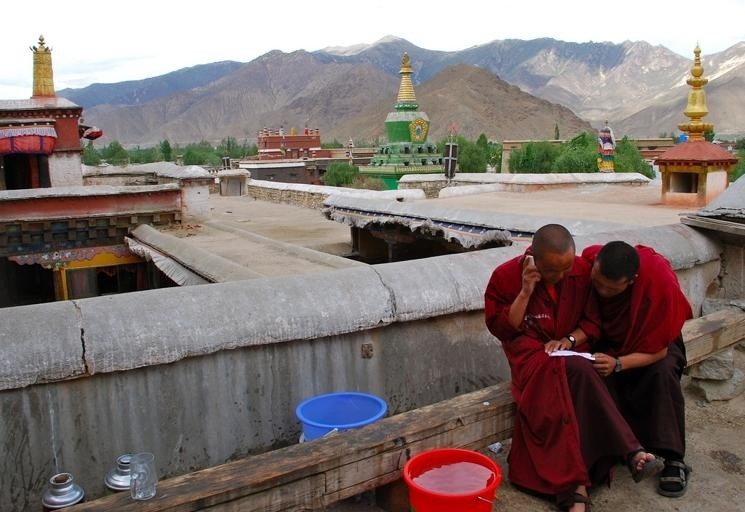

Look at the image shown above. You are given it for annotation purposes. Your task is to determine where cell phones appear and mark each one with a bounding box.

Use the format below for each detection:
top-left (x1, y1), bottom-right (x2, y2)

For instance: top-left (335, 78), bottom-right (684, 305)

top-left (526, 256), bottom-right (534, 265)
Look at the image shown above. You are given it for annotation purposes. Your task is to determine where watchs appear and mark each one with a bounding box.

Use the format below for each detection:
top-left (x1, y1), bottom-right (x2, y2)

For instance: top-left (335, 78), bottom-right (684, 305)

top-left (565, 334), bottom-right (577, 348)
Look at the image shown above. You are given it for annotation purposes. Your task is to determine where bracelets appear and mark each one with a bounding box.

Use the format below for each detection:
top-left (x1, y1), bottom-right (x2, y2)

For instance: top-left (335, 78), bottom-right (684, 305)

top-left (615, 357), bottom-right (623, 374)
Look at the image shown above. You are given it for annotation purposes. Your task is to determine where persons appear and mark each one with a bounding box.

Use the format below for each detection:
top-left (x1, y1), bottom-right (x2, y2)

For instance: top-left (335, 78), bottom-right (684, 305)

top-left (581, 242), bottom-right (693, 497)
top-left (485, 223), bottom-right (666, 511)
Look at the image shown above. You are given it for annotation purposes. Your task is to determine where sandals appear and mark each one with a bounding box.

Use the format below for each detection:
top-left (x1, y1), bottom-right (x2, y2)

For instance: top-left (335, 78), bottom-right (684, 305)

top-left (557, 490), bottom-right (592, 512)
top-left (629, 449), bottom-right (665, 482)
top-left (657, 457), bottom-right (688, 497)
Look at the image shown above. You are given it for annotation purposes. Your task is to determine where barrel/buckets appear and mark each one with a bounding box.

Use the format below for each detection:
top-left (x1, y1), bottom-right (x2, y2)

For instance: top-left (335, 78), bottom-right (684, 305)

top-left (294, 391), bottom-right (388, 446)
top-left (402, 447), bottom-right (504, 512)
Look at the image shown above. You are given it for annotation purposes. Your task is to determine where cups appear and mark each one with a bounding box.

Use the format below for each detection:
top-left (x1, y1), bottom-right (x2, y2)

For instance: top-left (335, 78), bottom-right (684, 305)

top-left (130, 451), bottom-right (159, 502)
